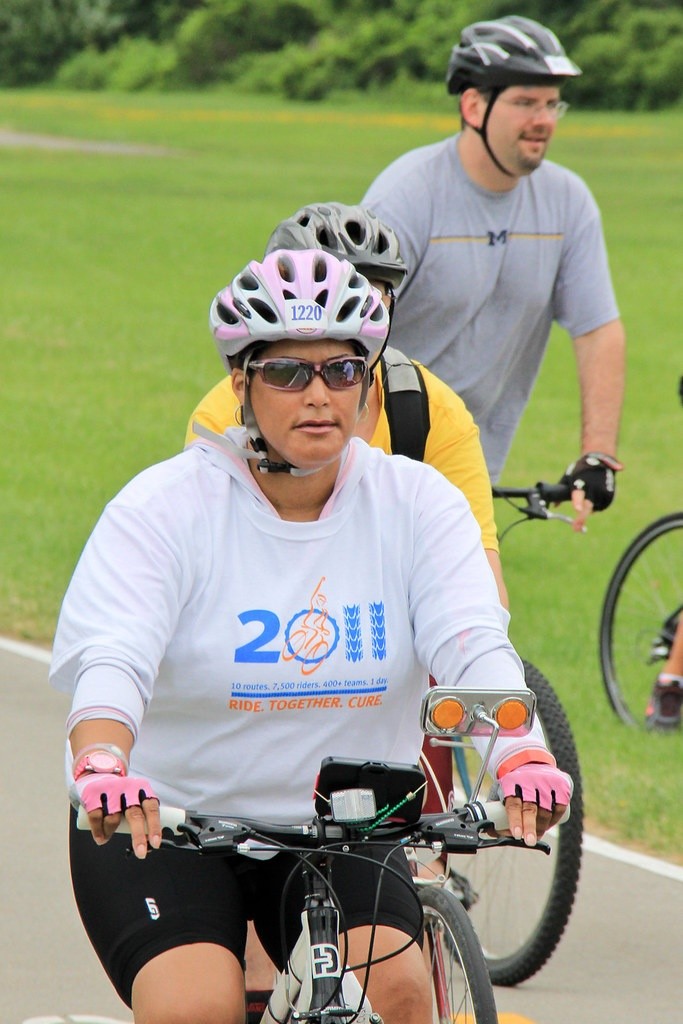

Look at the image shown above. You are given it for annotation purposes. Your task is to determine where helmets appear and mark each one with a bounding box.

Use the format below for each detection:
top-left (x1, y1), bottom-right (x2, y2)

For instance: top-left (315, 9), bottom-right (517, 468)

top-left (265, 202), bottom-right (409, 288)
top-left (211, 248), bottom-right (387, 369)
top-left (446, 15), bottom-right (579, 96)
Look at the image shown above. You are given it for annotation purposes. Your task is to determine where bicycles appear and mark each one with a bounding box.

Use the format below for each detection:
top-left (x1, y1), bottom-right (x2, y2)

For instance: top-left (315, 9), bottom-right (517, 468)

top-left (403, 745), bottom-right (504, 1024)
top-left (73, 684), bottom-right (571, 1024)
top-left (409, 478), bottom-right (596, 991)
top-left (600, 510), bottom-right (683, 736)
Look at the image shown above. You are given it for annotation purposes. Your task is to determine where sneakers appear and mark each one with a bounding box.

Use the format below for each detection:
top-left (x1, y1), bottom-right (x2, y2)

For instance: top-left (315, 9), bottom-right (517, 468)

top-left (649, 680), bottom-right (680, 734)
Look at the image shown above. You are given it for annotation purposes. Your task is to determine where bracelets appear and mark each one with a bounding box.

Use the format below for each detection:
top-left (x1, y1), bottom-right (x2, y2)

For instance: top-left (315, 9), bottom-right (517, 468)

top-left (75, 743), bottom-right (130, 774)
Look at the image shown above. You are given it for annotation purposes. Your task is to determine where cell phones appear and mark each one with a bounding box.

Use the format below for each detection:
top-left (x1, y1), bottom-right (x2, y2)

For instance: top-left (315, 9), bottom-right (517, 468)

top-left (420, 688), bottom-right (535, 738)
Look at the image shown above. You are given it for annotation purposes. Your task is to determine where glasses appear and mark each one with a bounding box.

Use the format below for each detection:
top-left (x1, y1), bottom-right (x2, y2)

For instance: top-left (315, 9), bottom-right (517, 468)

top-left (244, 353), bottom-right (371, 390)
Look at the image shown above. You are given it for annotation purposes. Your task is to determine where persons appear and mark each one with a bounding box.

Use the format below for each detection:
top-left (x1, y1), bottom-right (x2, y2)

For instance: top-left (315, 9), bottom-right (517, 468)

top-left (183, 202), bottom-right (512, 1022)
top-left (49, 251), bottom-right (574, 1023)
top-left (646, 610), bottom-right (682, 732)
top-left (360, 16), bottom-right (627, 532)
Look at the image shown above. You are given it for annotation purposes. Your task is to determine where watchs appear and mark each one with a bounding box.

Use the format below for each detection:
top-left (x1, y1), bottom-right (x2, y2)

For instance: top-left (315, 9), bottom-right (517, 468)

top-left (74, 751), bottom-right (126, 781)
